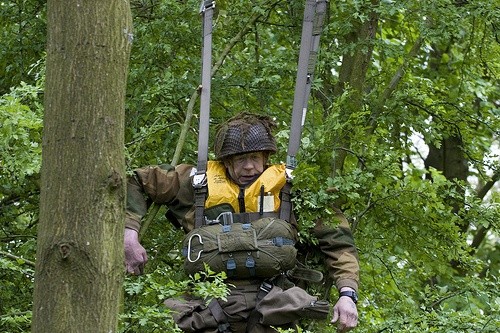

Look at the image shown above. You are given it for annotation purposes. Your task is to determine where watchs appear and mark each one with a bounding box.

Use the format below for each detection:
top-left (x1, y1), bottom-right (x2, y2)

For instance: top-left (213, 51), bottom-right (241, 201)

top-left (338, 291), bottom-right (358, 304)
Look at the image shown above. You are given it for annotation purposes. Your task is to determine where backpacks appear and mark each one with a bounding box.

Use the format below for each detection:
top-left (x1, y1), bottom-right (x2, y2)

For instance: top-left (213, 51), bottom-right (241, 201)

top-left (182, 166), bottom-right (300, 283)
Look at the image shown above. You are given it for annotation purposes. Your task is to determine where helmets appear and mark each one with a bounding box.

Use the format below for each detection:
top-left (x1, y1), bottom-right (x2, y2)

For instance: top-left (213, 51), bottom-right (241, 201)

top-left (213, 113), bottom-right (278, 187)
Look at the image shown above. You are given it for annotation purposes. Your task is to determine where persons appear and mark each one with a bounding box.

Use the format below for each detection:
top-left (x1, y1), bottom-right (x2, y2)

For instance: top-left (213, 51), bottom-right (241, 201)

top-left (123, 121), bottom-right (359, 333)
